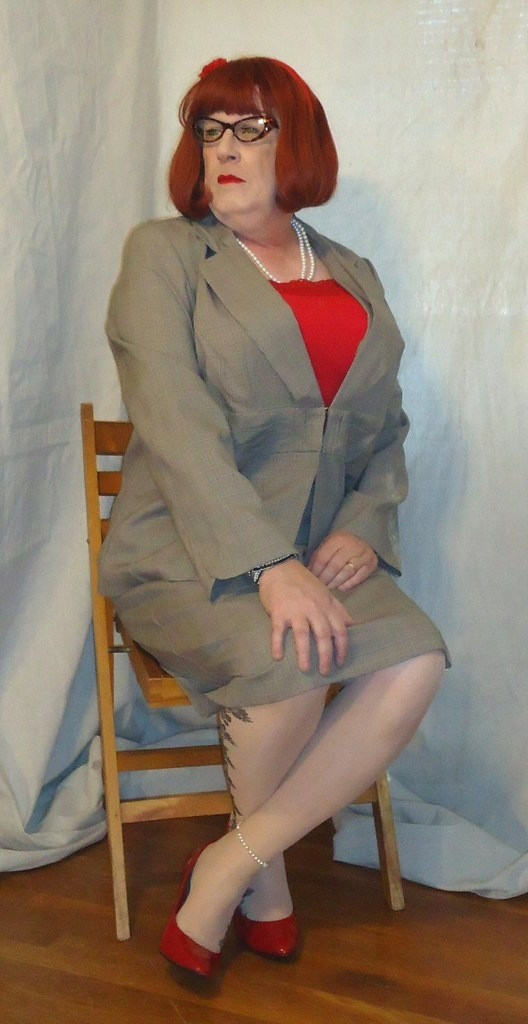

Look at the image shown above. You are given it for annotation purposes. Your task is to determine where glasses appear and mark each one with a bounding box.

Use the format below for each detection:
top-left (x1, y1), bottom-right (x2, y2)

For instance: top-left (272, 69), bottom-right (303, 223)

top-left (193, 115), bottom-right (280, 143)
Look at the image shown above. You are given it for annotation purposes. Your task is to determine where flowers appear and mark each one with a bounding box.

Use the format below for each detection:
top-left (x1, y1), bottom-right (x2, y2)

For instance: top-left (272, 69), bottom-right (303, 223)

top-left (199, 58), bottom-right (228, 79)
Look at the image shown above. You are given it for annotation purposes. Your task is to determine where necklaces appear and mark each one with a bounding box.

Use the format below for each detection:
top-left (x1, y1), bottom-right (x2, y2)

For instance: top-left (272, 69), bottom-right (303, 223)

top-left (237, 219), bottom-right (315, 283)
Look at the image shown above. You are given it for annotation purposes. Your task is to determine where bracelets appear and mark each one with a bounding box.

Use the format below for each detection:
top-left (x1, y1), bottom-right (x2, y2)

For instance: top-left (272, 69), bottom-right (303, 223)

top-left (252, 553), bottom-right (296, 582)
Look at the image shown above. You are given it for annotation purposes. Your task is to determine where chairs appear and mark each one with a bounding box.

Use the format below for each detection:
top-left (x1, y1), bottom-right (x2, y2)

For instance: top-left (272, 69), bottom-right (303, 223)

top-left (80, 402), bottom-right (406, 941)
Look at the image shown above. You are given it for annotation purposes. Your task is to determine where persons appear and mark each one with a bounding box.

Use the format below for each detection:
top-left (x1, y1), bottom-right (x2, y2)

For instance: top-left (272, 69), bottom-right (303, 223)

top-left (101, 57), bottom-right (450, 980)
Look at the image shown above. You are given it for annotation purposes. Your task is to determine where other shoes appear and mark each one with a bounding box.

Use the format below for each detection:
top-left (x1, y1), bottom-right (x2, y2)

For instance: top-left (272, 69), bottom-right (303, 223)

top-left (158, 838), bottom-right (226, 983)
top-left (225, 820), bottom-right (301, 962)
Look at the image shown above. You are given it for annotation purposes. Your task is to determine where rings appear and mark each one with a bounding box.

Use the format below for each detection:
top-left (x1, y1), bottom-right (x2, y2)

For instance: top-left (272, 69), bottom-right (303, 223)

top-left (349, 564), bottom-right (355, 570)
